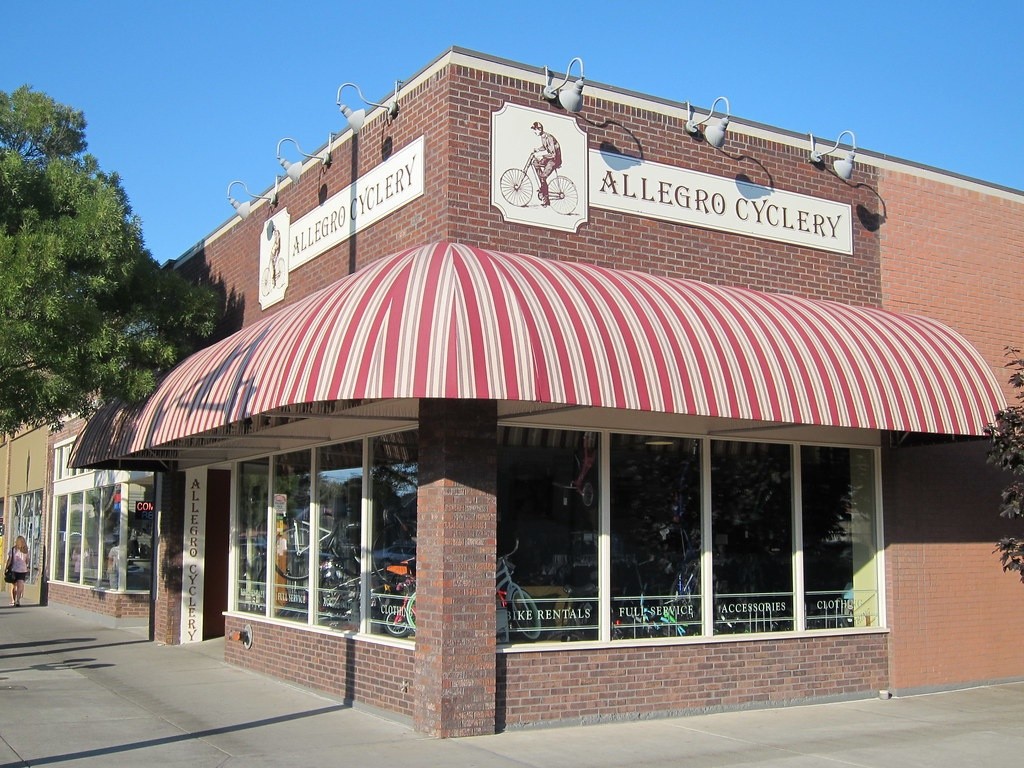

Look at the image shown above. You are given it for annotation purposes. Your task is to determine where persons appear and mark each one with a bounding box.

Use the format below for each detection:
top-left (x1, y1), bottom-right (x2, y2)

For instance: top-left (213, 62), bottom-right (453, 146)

top-left (108, 543), bottom-right (119, 590)
top-left (72, 540), bottom-right (88, 582)
top-left (6, 536), bottom-right (28, 607)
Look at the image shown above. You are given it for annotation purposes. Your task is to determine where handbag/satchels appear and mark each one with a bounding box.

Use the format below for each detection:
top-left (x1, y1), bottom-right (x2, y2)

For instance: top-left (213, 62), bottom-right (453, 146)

top-left (4, 570), bottom-right (16, 583)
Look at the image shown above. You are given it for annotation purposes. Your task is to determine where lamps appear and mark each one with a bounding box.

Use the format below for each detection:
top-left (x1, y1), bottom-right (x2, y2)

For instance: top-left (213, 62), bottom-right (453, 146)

top-left (543, 57), bottom-right (585, 112)
top-left (227, 175), bottom-right (282, 222)
top-left (809, 131), bottom-right (857, 180)
top-left (685, 96), bottom-right (730, 148)
top-left (277, 132), bottom-right (336, 185)
top-left (337, 80), bottom-right (403, 135)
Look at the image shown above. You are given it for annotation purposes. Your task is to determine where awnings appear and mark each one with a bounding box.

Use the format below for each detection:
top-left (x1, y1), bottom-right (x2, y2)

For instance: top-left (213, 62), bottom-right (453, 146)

top-left (66, 244), bottom-right (1013, 471)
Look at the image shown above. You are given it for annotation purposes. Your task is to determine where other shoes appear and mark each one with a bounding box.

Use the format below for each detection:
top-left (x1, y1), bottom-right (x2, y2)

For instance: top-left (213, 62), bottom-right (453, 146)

top-left (8, 601), bottom-right (21, 608)
